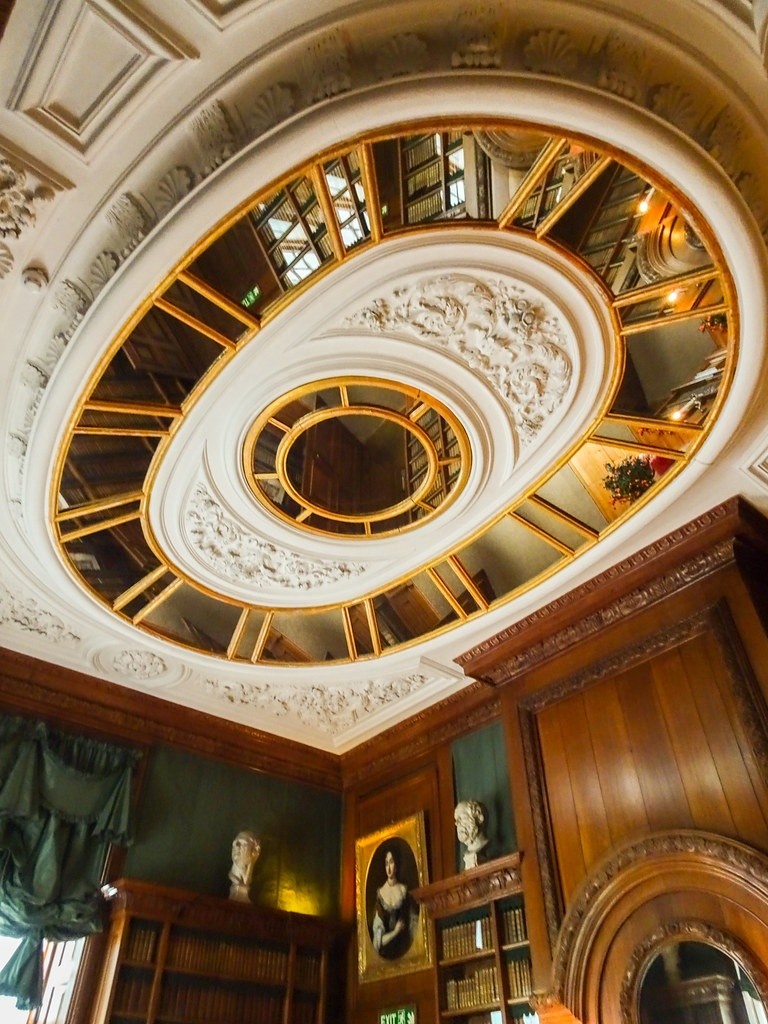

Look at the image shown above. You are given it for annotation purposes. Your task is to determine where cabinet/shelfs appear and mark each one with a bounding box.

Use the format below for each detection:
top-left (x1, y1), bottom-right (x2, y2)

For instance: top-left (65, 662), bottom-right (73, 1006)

top-left (397, 132), bottom-right (476, 224)
top-left (237, 136), bottom-right (371, 293)
top-left (251, 400), bottom-right (314, 519)
top-left (410, 851), bottom-right (533, 1024)
top-left (514, 145), bottom-right (593, 231)
top-left (406, 398), bottom-right (462, 524)
top-left (578, 161), bottom-right (655, 287)
top-left (55, 311), bottom-right (201, 621)
top-left (87, 879), bottom-right (354, 1024)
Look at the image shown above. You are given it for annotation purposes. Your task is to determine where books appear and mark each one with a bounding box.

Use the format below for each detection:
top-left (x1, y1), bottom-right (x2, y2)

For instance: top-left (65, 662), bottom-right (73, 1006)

top-left (514, 144), bottom-right (652, 292)
top-left (440, 909), bottom-right (532, 1024)
top-left (112, 925), bottom-right (320, 1024)
top-left (59, 280), bottom-right (218, 616)
top-left (400, 133), bottom-right (444, 222)
top-left (249, 149), bottom-right (376, 289)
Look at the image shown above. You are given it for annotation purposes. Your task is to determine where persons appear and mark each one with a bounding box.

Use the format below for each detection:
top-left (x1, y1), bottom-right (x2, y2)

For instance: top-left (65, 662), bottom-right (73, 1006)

top-left (453, 800), bottom-right (491, 870)
top-left (228, 830), bottom-right (265, 904)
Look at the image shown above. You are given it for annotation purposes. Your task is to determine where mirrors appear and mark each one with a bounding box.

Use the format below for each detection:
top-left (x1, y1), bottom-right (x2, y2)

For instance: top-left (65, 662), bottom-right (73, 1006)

top-left (619, 920), bottom-right (768, 1024)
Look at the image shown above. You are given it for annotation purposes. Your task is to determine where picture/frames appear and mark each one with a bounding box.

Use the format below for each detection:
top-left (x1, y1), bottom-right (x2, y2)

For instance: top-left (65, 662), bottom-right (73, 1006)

top-left (354, 812), bottom-right (436, 984)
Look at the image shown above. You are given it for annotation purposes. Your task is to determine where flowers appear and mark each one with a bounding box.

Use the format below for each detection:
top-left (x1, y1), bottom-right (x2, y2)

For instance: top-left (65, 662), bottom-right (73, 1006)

top-left (697, 313), bottom-right (727, 334)
top-left (603, 453), bottom-right (657, 510)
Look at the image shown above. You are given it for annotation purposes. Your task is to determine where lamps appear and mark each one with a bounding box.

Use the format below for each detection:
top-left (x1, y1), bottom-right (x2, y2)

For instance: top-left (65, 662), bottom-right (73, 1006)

top-left (675, 392), bottom-right (705, 419)
